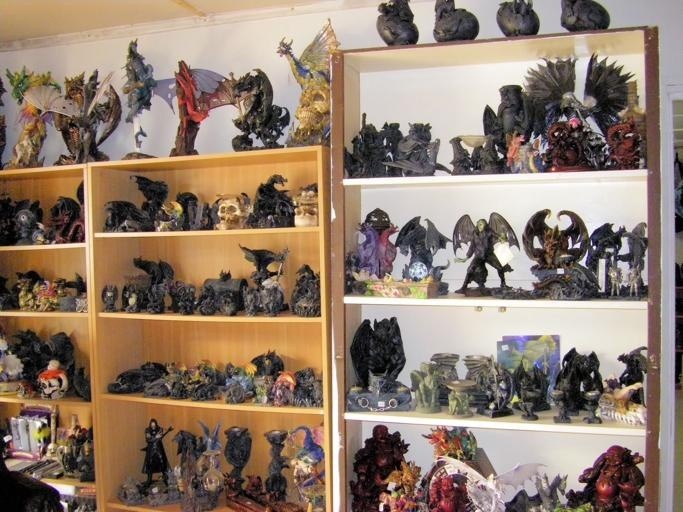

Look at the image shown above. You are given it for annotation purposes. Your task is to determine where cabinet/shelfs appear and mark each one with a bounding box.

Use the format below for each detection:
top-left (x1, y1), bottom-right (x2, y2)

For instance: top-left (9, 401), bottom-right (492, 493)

top-left (326, 25), bottom-right (665, 512)
top-left (1, 144), bottom-right (332, 512)
